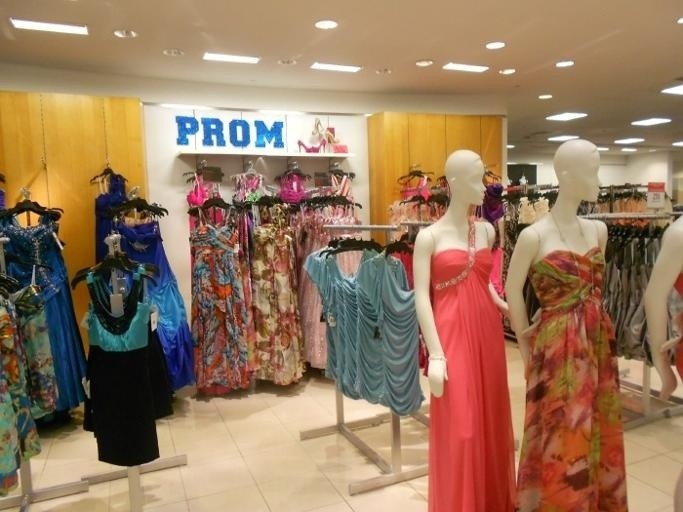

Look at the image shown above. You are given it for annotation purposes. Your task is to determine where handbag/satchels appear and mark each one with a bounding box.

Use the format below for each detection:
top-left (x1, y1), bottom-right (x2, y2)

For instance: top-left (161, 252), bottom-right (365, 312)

top-left (517, 197), bottom-right (549, 225)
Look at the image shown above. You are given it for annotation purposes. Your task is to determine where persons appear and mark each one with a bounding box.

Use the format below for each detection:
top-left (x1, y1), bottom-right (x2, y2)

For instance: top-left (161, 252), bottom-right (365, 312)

top-left (504, 139), bottom-right (631, 511)
top-left (644, 213), bottom-right (683, 512)
top-left (412, 148), bottom-right (519, 512)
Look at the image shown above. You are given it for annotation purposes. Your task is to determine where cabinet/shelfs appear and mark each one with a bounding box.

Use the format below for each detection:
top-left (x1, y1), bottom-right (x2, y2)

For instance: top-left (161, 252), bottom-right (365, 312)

top-left (368, 112), bottom-right (503, 249)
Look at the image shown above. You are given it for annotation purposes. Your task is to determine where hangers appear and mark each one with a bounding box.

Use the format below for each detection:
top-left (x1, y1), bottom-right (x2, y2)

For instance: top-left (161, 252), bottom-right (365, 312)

top-left (483, 163), bottom-right (561, 210)
top-left (598, 183), bottom-right (646, 202)
top-left (604, 214), bottom-right (671, 253)
top-left (183, 160), bottom-right (361, 217)
top-left (88, 157), bottom-right (170, 219)
top-left (71, 231), bottom-right (160, 291)
top-left (398, 165), bottom-right (450, 211)
top-left (319, 225), bottom-right (413, 259)
top-left (0, 271), bottom-right (25, 301)
top-left (0, 232), bottom-right (55, 273)
top-left (0, 187), bottom-right (65, 223)
